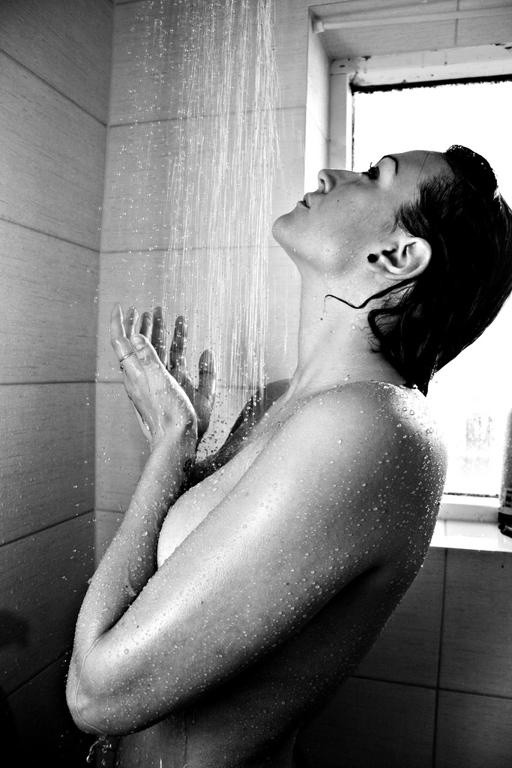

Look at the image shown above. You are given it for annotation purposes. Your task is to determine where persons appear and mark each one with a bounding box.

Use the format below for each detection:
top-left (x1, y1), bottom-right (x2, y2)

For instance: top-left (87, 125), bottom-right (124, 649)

top-left (64, 145), bottom-right (512, 767)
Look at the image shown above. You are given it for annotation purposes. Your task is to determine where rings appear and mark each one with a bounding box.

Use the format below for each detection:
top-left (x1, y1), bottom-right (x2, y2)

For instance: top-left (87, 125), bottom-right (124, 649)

top-left (119, 352), bottom-right (133, 363)
